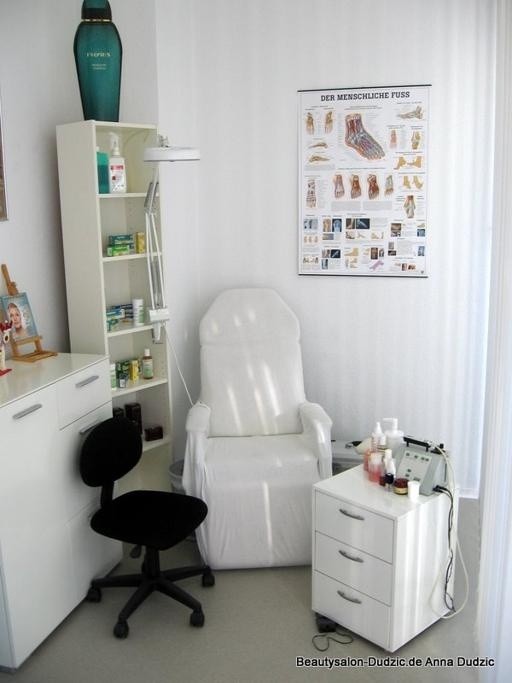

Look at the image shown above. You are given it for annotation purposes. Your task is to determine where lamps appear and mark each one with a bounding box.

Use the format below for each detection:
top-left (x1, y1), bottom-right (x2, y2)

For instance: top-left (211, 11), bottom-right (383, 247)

top-left (139, 139), bottom-right (206, 422)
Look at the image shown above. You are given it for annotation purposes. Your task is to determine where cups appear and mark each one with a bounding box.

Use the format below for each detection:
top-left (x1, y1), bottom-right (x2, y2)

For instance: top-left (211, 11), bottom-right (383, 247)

top-left (407, 481), bottom-right (420, 497)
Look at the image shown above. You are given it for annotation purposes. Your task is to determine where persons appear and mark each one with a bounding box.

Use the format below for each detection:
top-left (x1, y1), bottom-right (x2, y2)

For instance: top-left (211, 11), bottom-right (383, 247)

top-left (3, 302), bottom-right (30, 341)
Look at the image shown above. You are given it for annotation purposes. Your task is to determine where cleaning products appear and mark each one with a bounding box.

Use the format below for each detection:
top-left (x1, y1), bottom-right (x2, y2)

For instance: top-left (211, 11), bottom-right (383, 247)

top-left (95, 143), bottom-right (110, 194)
top-left (108, 132), bottom-right (127, 194)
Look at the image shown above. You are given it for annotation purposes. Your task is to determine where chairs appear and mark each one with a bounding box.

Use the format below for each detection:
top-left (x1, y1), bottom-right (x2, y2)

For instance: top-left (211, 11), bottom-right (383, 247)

top-left (181, 284), bottom-right (339, 570)
top-left (77, 413), bottom-right (215, 630)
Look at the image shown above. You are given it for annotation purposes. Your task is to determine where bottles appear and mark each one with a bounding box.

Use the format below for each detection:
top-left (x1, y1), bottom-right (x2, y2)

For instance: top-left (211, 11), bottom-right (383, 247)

top-left (141, 348), bottom-right (154, 379)
top-left (362, 421), bottom-right (407, 496)
top-left (73, 0), bottom-right (122, 122)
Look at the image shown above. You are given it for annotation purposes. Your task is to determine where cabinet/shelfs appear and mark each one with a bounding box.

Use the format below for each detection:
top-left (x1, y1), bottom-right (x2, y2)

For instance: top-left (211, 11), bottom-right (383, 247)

top-left (51, 118), bottom-right (179, 555)
top-left (0, 349), bottom-right (126, 631)
top-left (309, 462), bottom-right (461, 658)
